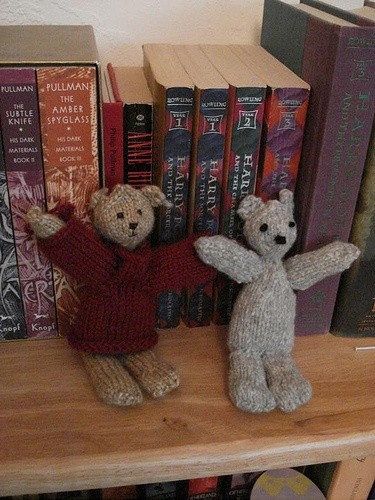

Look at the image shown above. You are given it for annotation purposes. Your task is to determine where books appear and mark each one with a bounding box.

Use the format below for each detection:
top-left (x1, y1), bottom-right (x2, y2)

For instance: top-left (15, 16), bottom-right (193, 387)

top-left (260, 0), bottom-right (375, 338)
top-left (0, 463), bottom-right (337, 500)
top-left (0, 25), bottom-right (100, 343)
top-left (102, 45), bottom-right (312, 330)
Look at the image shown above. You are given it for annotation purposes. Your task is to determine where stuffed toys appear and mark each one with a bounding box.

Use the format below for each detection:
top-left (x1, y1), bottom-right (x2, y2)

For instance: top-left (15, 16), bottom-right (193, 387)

top-left (27, 184), bottom-right (217, 406)
top-left (194, 188), bottom-right (360, 414)
